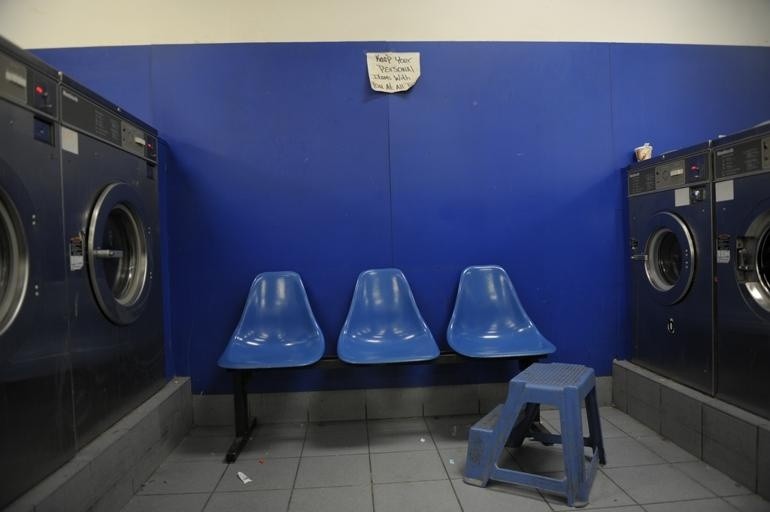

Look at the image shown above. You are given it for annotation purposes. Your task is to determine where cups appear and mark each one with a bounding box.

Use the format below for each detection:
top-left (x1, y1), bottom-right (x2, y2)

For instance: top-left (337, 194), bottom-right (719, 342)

top-left (634, 146), bottom-right (653, 163)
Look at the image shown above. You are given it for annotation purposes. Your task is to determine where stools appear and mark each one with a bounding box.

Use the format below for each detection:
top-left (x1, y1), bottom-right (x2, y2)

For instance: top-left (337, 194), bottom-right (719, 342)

top-left (461, 358), bottom-right (608, 505)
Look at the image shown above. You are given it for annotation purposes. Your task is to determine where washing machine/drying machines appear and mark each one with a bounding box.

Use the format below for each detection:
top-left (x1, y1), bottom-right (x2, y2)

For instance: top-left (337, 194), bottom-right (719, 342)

top-left (624, 118), bottom-right (769, 423)
top-left (0, 33), bottom-right (168, 512)
